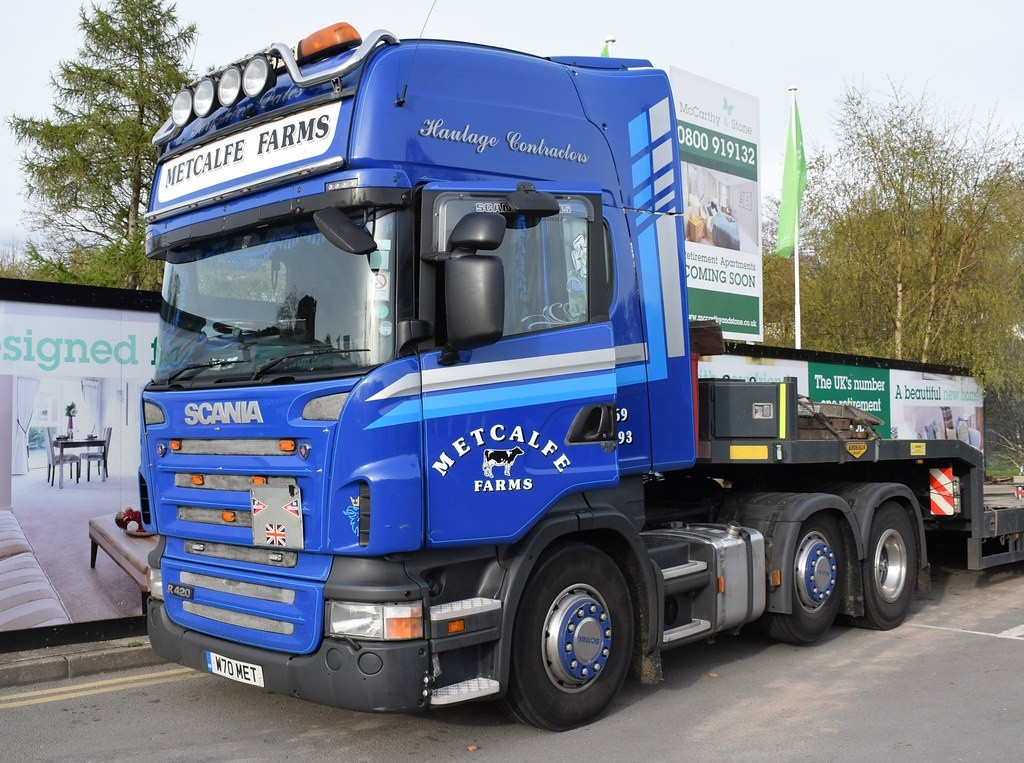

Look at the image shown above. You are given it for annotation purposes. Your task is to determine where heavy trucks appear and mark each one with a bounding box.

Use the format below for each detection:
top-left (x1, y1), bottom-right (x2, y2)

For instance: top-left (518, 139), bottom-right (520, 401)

top-left (136, 21), bottom-right (1024, 729)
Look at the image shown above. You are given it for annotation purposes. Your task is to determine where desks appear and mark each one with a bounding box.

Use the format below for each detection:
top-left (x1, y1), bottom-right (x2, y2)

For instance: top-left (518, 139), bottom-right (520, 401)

top-left (89, 512), bottom-right (160, 615)
top-left (52, 439), bottom-right (106, 489)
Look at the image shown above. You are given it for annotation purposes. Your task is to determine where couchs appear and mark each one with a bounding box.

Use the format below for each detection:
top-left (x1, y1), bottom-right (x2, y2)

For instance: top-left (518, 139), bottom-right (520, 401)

top-left (0, 509), bottom-right (73, 631)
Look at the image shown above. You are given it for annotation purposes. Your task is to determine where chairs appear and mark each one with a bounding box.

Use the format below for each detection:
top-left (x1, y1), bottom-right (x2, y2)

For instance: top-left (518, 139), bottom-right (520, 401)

top-left (78, 427), bottom-right (113, 482)
top-left (44, 427), bottom-right (81, 487)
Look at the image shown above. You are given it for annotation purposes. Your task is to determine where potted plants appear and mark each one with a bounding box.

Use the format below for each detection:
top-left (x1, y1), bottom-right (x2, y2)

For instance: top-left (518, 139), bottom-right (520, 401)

top-left (65, 402), bottom-right (77, 439)
